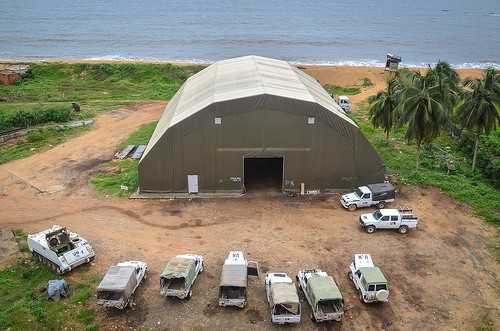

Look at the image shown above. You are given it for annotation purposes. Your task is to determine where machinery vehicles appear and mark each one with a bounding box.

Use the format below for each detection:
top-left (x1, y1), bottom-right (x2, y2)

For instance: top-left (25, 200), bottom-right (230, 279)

top-left (27, 224), bottom-right (95, 275)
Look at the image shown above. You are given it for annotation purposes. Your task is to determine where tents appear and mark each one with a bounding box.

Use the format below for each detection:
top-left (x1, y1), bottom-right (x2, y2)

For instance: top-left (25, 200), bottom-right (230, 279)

top-left (128, 54), bottom-right (385, 203)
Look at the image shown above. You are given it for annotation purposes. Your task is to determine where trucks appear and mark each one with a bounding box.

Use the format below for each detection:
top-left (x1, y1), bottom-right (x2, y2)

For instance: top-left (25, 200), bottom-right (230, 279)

top-left (219, 251), bottom-right (259, 308)
top-left (348, 254), bottom-right (389, 304)
top-left (266, 273), bottom-right (301, 326)
top-left (340, 183), bottom-right (395, 211)
top-left (297, 268), bottom-right (345, 323)
top-left (159, 254), bottom-right (204, 300)
top-left (97, 260), bottom-right (148, 310)
top-left (331, 94), bottom-right (350, 112)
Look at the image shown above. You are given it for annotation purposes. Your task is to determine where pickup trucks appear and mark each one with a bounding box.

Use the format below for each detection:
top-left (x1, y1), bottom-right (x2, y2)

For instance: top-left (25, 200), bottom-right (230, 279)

top-left (359, 209), bottom-right (418, 233)
